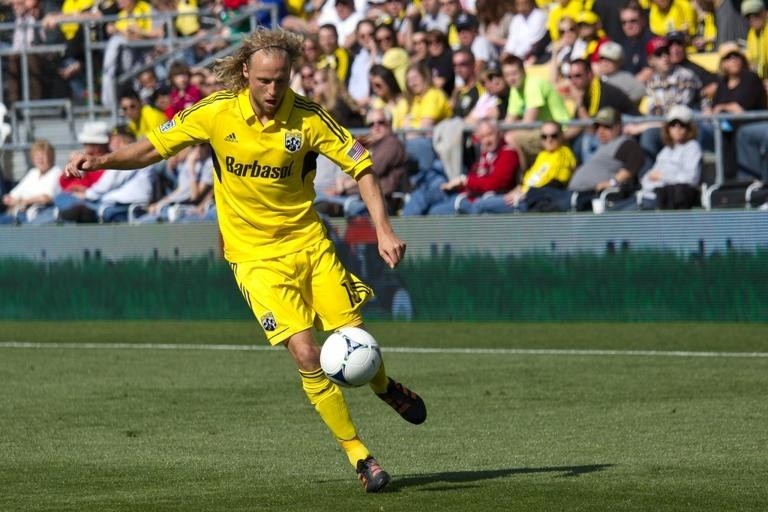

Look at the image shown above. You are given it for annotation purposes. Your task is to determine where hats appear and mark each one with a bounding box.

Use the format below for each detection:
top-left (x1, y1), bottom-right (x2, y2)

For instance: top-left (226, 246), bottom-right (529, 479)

top-left (740, 0), bottom-right (764, 16)
top-left (646, 35), bottom-right (670, 53)
top-left (599, 42), bottom-right (624, 61)
top-left (718, 38), bottom-right (739, 59)
top-left (594, 107), bottom-right (619, 128)
top-left (666, 106), bottom-right (692, 125)
top-left (78, 122), bottom-right (110, 144)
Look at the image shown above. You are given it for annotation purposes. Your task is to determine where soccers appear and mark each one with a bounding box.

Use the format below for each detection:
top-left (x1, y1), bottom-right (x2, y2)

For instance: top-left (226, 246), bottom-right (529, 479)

top-left (319, 327), bottom-right (381, 387)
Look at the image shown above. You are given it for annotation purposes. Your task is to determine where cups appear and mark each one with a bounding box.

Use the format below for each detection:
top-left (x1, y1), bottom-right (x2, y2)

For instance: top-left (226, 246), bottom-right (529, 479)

top-left (167, 206), bottom-right (183, 222)
top-left (591, 198), bottom-right (605, 216)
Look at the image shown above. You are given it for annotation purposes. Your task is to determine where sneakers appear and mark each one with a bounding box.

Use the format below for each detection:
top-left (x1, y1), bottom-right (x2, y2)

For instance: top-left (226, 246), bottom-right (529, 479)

top-left (374, 376), bottom-right (426, 425)
top-left (355, 455), bottom-right (391, 493)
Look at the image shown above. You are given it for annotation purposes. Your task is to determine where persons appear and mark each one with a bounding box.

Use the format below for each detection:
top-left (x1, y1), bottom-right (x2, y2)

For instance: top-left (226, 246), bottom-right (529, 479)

top-left (0, 1), bottom-right (768, 218)
top-left (62, 21), bottom-right (428, 494)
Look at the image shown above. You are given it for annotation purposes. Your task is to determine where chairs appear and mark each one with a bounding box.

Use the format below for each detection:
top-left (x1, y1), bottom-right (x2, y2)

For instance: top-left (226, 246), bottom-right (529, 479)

top-left (12, 121), bottom-right (768, 225)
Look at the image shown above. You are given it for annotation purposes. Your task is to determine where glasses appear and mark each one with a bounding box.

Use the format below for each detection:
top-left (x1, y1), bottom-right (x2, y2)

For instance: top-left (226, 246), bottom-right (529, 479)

top-left (541, 132), bottom-right (558, 140)
top-left (366, 120), bottom-right (384, 127)
